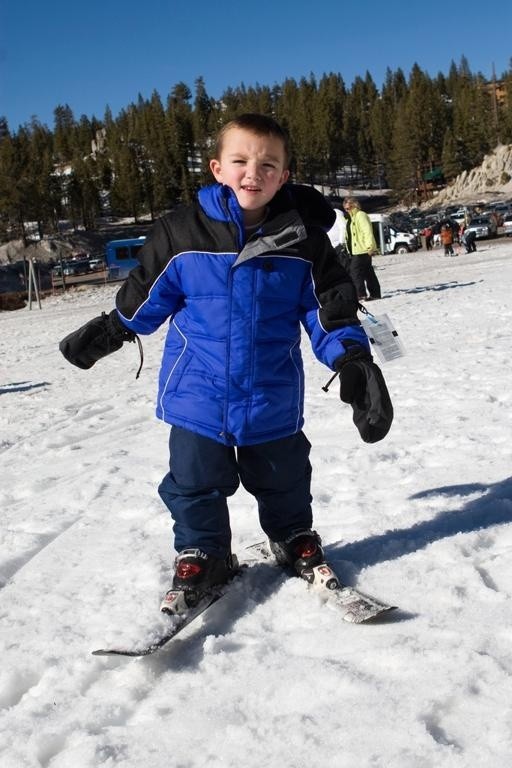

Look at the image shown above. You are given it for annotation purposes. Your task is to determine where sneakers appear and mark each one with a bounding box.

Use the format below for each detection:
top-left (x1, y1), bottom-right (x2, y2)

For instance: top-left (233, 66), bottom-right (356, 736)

top-left (273, 527), bottom-right (325, 571)
top-left (172, 546), bottom-right (233, 591)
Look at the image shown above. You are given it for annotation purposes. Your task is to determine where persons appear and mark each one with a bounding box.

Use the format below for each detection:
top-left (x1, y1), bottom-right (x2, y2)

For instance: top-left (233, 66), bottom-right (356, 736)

top-left (60, 112), bottom-right (393, 620)
top-left (342, 195), bottom-right (382, 301)
top-left (326, 196), bottom-right (350, 274)
top-left (420, 221), bottom-right (476, 257)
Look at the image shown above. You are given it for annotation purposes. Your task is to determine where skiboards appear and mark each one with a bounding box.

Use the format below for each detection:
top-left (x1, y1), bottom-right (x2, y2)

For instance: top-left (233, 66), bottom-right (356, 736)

top-left (91, 540), bottom-right (399, 654)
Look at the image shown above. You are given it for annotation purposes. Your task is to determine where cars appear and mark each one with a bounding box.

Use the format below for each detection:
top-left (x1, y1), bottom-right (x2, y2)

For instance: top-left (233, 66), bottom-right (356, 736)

top-left (52, 254), bottom-right (106, 276)
top-left (409, 200), bottom-right (512, 239)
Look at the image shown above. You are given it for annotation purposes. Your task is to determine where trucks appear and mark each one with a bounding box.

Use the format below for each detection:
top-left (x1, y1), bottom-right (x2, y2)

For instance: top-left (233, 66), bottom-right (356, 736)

top-left (366, 214), bottom-right (420, 254)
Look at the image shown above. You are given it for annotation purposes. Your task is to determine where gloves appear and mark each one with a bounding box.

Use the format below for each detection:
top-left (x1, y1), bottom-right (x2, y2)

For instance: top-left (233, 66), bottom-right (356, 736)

top-left (57, 308), bottom-right (137, 370)
top-left (333, 333), bottom-right (394, 444)
